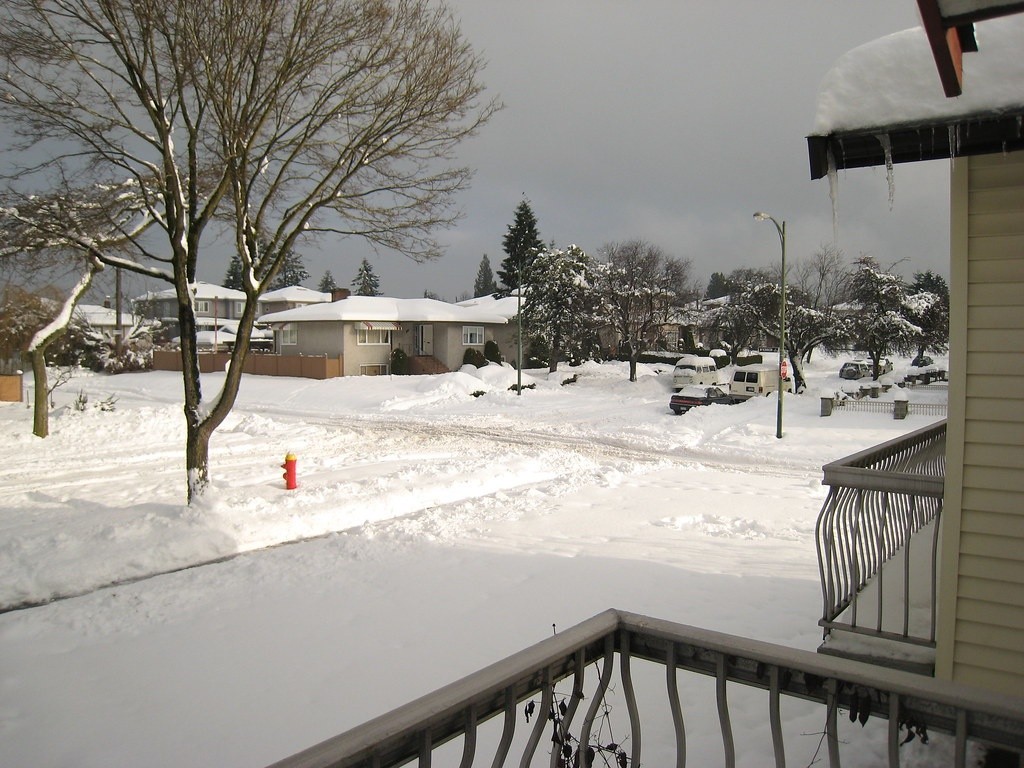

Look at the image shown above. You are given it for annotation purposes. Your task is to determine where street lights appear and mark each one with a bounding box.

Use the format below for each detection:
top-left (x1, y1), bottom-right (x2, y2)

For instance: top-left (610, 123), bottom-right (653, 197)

top-left (752, 210), bottom-right (786, 438)
top-left (516, 246), bottom-right (537, 394)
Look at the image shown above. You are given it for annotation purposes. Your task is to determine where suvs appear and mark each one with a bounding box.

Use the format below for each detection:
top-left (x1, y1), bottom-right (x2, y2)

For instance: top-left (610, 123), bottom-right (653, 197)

top-left (839, 356), bottom-right (894, 381)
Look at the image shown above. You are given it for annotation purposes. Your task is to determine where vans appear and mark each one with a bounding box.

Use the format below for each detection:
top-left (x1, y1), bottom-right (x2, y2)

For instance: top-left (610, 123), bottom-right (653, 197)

top-left (727, 364), bottom-right (793, 405)
top-left (672, 356), bottom-right (718, 391)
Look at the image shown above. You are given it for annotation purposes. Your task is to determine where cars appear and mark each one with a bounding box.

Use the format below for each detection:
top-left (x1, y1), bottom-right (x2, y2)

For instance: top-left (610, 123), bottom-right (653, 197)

top-left (912, 355), bottom-right (934, 367)
top-left (669, 385), bottom-right (732, 415)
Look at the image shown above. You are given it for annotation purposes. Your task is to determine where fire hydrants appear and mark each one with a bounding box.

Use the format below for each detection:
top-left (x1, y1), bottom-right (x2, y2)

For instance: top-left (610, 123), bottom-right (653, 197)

top-left (281, 451), bottom-right (299, 490)
